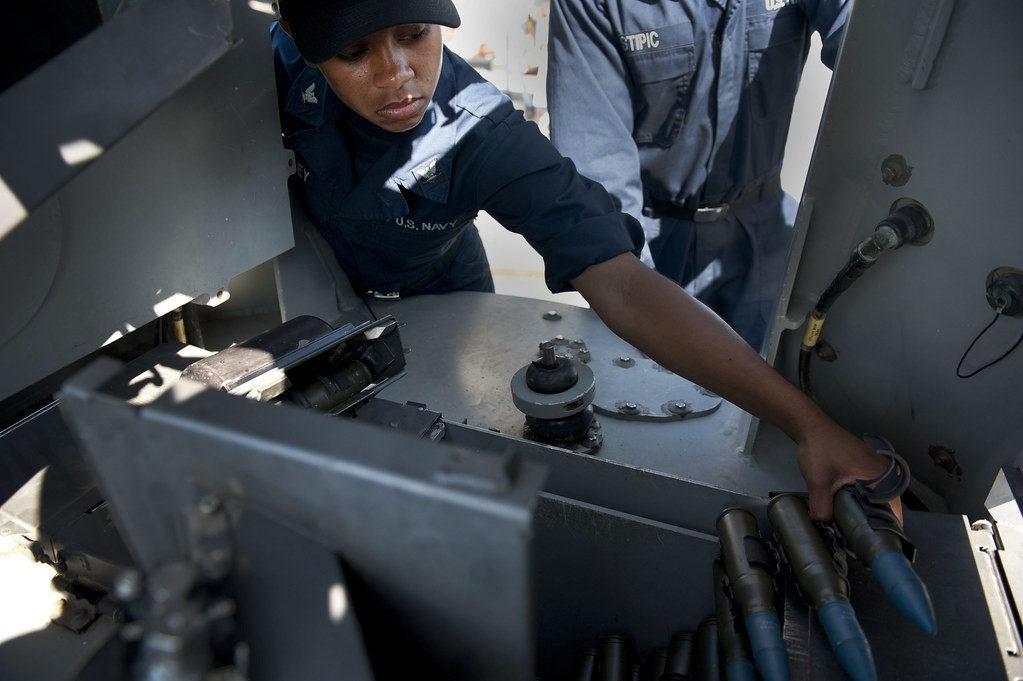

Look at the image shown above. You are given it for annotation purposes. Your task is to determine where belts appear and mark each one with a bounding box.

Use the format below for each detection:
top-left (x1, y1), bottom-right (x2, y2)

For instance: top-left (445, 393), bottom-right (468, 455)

top-left (643, 174), bottom-right (781, 223)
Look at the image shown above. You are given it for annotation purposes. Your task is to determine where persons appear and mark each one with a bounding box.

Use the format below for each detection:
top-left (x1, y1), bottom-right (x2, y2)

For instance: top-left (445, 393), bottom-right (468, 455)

top-left (267, 0), bottom-right (903, 554)
top-left (547, 0), bottom-right (852, 356)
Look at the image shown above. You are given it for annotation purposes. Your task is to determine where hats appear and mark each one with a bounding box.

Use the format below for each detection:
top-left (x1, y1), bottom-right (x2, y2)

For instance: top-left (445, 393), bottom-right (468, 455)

top-left (279, 0), bottom-right (461, 65)
